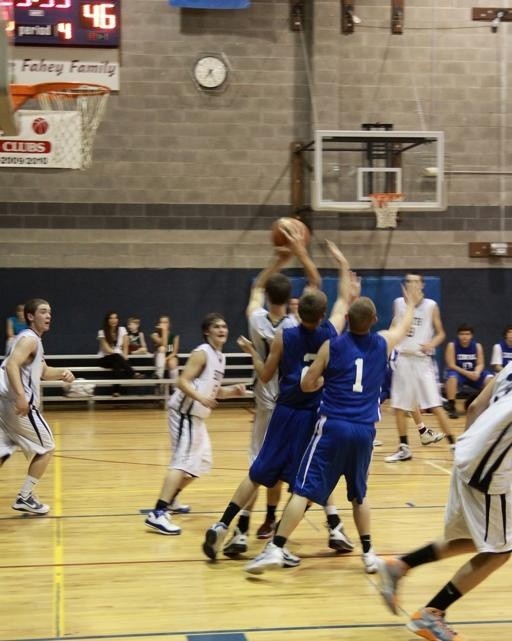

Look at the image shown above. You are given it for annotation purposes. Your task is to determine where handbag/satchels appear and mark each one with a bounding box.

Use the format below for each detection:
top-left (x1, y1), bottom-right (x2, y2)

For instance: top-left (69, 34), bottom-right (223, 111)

top-left (64, 380), bottom-right (98, 398)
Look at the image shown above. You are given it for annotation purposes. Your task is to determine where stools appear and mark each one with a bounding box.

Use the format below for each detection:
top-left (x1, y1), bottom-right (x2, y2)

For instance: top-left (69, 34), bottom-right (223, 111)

top-left (457, 384), bottom-right (478, 399)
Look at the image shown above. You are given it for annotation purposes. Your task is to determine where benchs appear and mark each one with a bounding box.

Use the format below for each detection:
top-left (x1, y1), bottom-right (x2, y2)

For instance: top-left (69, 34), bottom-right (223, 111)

top-left (1, 353), bottom-right (261, 411)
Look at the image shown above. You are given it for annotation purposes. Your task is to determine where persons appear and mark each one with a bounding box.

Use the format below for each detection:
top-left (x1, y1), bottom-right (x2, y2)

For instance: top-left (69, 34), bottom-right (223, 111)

top-left (96, 312), bottom-right (181, 399)
top-left (146, 312), bottom-right (245, 536)
top-left (359, 389), bottom-right (512, 640)
top-left (0, 298), bottom-right (75, 513)
top-left (441, 323), bottom-right (510, 418)
top-left (387, 271), bottom-right (458, 462)
top-left (200, 237), bottom-right (354, 560)
top-left (243, 280), bottom-right (425, 575)
top-left (289, 297), bottom-right (299, 313)
top-left (220, 222), bottom-right (322, 569)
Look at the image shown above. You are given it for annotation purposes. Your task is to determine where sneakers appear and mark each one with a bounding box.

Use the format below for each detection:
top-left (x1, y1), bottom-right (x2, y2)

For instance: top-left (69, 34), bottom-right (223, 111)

top-left (387, 442), bottom-right (412, 463)
top-left (261, 539), bottom-right (302, 567)
top-left (406, 607), bottom-right (463, 641)
top-left (222, 528), bottom-right (250, 554)
top-left (167, 499), bottom-right (190, 514)
top-left (243, 542), bottom-right (291, 575)
top-left (377, 556), bottom-right (410, 617)
top-left (362, 548), bottom-right (379, 573)
top-left (11, 492), bottom-right (50, 515)
top-left (419, 428), bottom-right (447, 445)
top-left (256, 516), bottom-right (276, 539)
top-left (144, 510), bottom-right (182, 536)
top-left (321, 519), bottom-right (356, 553)
top-left (202, 522), bottom-right (229, 560)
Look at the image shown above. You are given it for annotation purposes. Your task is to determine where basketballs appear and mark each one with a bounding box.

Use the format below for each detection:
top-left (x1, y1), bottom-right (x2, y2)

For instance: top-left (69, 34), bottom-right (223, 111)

top-left (273, 217), bottom-right (310, 250)
top-left (32, 118), bottom-right (48, 134)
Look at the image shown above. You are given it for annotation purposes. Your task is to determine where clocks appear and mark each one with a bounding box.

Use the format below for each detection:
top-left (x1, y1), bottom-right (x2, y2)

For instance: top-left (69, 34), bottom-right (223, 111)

top-left (194, 55), bottom-right (228, 90)
